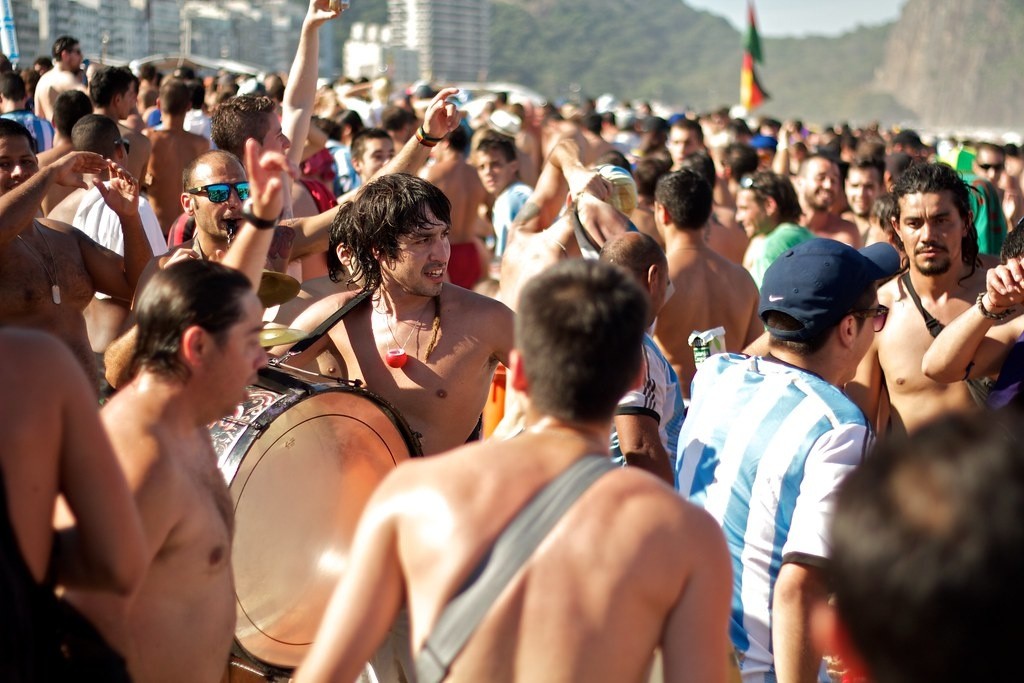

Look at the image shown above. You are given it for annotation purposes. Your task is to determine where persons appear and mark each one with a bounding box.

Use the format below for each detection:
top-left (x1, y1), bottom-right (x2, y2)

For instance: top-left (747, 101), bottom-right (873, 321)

top-left (0, 0), bottom-right (1024, 683)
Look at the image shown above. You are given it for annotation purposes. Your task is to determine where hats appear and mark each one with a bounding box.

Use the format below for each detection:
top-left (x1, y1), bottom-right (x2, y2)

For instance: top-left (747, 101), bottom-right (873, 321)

top-left (642, 117), bottom-right (670, 131)
top-left (596, 93), bottom-right (619, 116)
top-left (756, 237), bottom-right (899, 344)
top-left (486, 108), bottom-right (521, 138)
top-left (893, 127), bottom-right (929, 151)
top-left (614, 108), bottom-right (636, 129)
top-left (173, 65), bottom-right (195, 78)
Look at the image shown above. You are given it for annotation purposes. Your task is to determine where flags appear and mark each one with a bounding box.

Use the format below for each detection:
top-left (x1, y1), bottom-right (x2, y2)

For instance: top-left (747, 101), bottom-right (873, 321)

top-left (745, 5), bottom-right (761, 60)
top-left (740, 54), bottom-right (769, 113)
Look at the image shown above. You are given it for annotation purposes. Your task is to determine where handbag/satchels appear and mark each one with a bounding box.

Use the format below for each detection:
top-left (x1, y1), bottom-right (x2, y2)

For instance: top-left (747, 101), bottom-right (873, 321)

top-left (899, 269), bottom-right (997, 412)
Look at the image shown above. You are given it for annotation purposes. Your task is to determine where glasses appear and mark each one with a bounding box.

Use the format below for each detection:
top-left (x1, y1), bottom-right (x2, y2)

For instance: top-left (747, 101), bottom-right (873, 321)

top-left (189, 180), bottom-right (250, 203)
top-left (115, 137), bottom-right (130, 154)
top-left (845, 304), bottom-right (889, 332)
top-left (976, 157), bottom-right (1002, 171)
top-left (72, 48), bottom-right (81, 54)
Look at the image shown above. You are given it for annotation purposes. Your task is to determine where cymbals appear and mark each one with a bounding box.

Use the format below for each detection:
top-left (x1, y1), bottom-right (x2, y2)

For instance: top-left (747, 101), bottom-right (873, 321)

top-left (261, 328), bottom-right (313, 352)
top-left (256, 269), bottom-right (305, 308)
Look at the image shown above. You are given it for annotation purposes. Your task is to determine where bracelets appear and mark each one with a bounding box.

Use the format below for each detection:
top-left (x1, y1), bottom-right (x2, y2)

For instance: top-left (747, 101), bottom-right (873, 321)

top-left (415, 124), bottom-right (442, 147)
top-left (976, 292), bottom-right (1016, 319)
top-left (242, 198), bottom-right (278, 229)
top-left (988, 294), bottom-right (1015, 308)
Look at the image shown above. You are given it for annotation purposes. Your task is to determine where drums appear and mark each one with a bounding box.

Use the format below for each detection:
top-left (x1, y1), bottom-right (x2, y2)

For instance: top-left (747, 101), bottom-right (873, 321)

top-left (201, 368), bottom-right (427, 673)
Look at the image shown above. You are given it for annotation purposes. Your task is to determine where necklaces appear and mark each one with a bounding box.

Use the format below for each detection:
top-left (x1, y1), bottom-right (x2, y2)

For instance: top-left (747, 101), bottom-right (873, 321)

top-left (17, 223), bottom-right (61, 305)
top-left (669, 247), bottom-right (708, 250)
top-left (543, 228), bottom-right (568, 258)
top-left (382, 296), bottom-right (425, 368)
